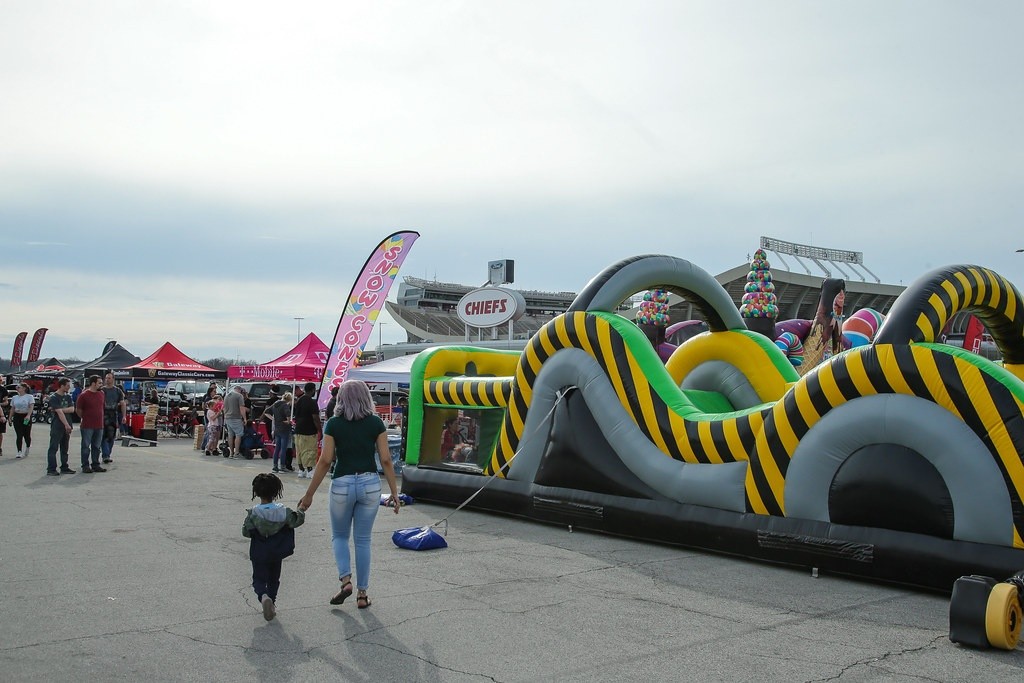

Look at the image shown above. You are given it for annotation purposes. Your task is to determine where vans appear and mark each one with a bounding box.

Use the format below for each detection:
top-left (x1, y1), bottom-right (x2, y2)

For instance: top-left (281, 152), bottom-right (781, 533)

top-left (159, 380), bottom-right (225, 406)
top-left (320, 390), bottom-right (409, 423)
top-left (271, 380), bottom-right (320, 399)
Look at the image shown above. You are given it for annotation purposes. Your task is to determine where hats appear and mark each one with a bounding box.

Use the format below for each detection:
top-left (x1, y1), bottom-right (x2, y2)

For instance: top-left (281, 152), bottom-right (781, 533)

top-left (270, 385), bottom-right (280, 395)
top-left (234, 386), bottom-right (243, 394)
top-left (241, 388), bottom-right (248, 394)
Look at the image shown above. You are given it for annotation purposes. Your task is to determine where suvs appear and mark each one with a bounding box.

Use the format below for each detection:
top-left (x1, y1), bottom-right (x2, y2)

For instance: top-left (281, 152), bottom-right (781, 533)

top-left (224, 382), bottom-right (293, 419)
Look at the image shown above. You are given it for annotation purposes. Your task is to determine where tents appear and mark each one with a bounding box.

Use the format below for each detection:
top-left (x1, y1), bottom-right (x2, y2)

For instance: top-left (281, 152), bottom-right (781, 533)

top-left (347, 353), bottom-right (420, 423)
top-left (222, 332), bottom-right (330, 448)
top-left (64, 344), bottom-right (141, 390)
top-left (2, 357), bottom-right (69, 394)
top-left (128, 342), bottom-right (226, 431)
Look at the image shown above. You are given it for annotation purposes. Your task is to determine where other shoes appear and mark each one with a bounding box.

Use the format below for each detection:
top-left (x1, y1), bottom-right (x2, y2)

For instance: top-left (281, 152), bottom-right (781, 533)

top-left (229, 452), bottom-right (245, 459)
top-left (286, 465), bottom-right (295, 471)
top-left (91, 465), bottom-right (107, 472)
top-left (83, 465), bottom-right (93, 473)
top-left (103, 457), bottom-right (113, 464)
top-left (25, 446), bottom-right (30, 456)
top-left (61, 468), bottom-right (75, 474)
top-left (261, 594), bottom-right (276, 621)
top-left (47, 470), bottom-right (59, 476)
top-left (272, 468), bottom-right (279, 473)
top-left (16, 451), bottom-right (23, 457)
top-left (0, 449), bottom-right (2, 456)
top-left (278, 468), bottom-right (291, 473)
top-left (206, 450), bottom-right (219, 456)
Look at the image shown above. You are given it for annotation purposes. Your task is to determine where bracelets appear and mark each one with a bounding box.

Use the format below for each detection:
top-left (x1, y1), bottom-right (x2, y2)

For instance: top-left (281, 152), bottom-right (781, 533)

top-left (1, 416), bottom-right (4, 417)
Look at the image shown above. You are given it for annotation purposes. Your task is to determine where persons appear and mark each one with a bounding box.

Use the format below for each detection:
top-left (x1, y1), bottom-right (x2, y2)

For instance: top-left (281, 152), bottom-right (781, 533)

top-left (0, 372), bottom-right (339, 479)
top-left (297, 380), bottom-right (400, 609)
top-left (242, 472), bottom-right (309, 622)
top-left (441, 417), bottom-right (464, 463)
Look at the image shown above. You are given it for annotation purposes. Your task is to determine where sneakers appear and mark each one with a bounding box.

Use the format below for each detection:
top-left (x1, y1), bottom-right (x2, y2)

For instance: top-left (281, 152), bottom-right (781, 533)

top-left (297, 469), bottom-right (307, 478)
top-left (306, 470), bottom-right (314, 478)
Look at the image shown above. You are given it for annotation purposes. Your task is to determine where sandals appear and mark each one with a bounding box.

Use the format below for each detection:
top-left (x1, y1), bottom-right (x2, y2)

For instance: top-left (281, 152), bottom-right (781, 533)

top-left (330, 582), bottom-right (353, 604)
top-left (357, 596), bottom-right (371, 609)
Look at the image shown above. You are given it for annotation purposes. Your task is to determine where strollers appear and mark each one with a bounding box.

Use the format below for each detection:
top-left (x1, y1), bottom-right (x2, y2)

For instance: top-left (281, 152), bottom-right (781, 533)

top-left (222, 418), bottom-right (270, 460)
